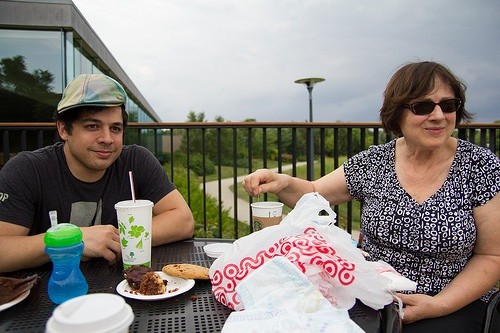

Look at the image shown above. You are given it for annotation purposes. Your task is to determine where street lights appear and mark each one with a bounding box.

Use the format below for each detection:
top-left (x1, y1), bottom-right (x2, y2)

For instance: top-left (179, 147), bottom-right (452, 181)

top-left (295, 77), bottom-right (326, 181)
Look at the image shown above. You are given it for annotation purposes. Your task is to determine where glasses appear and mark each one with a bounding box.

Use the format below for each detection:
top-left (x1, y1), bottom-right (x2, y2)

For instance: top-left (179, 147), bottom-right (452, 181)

top-left (404, 98), bottom-right (462, 115)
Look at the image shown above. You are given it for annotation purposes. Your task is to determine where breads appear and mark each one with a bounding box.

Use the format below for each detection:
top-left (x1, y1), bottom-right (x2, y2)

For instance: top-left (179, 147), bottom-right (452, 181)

top-left (125, 265), bottom-right (154, 289)
top-left (141, 272), bottom-right (165, 295)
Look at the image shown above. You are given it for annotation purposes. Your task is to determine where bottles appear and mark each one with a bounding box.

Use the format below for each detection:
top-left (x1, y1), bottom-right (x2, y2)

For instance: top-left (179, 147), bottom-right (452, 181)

top-left (44, 210), bottom-right (89, 305)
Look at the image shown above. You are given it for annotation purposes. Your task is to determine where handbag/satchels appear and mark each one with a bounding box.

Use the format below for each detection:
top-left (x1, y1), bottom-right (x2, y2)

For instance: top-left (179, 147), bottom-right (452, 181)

top-left (208, 193), bottom-right (393, 310)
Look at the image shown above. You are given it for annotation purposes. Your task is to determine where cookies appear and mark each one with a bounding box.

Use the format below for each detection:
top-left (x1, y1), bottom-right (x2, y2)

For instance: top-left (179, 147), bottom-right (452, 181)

top-left (162, 263), bottom-right (211, 280)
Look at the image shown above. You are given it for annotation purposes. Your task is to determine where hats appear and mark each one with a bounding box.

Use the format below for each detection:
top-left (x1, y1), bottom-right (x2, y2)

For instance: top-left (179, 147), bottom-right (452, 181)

top-left (57, 73), bottom-right (127, 114)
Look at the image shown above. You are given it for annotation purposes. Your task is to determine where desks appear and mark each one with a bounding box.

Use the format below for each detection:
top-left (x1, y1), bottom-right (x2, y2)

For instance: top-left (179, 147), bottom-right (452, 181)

top-left (0, 238), bottom-right (401, 333)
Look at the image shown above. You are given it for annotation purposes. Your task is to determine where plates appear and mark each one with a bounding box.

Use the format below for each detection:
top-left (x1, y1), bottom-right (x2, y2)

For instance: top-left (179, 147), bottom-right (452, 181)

top-left (0, 277), bottom-right (31, 313)
top-left (116, 271), bottom-right (195, 302)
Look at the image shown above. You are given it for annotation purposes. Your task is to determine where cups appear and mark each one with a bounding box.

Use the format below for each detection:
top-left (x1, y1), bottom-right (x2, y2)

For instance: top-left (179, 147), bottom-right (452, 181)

top-left (45, 293), bottom-right (135, 333)
top-left (250, 201), bottom-right (284, 232)
top-left (114, 200), bottom-right (155, 270)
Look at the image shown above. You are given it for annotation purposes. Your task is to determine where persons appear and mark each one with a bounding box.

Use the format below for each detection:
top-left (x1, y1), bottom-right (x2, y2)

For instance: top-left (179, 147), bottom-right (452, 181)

top-left (0, 74), bottom-right (195, 272)
top-left (242, 62), bottom-right (500, 333)
